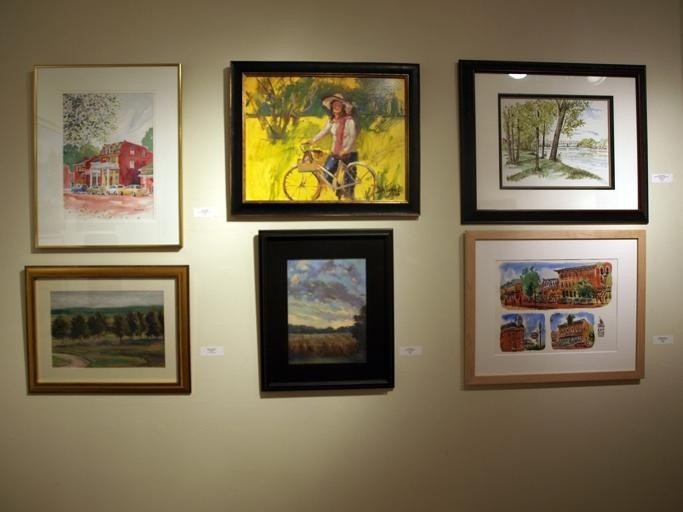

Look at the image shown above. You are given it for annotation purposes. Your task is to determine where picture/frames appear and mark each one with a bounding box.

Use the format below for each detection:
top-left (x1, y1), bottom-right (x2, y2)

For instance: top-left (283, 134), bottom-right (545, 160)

top-left (462, 229), bottom-right (648, 388)
top-left (258, 227), bottom-right (395, 392)
top-left (28, 62), bottom-right (183, 253)
top-left (458, 59), bottom-right (648, 225)
top-left (24, 265), bottom-right (192, 395)
top-left (226, 60), bottom-right (420, 219)
